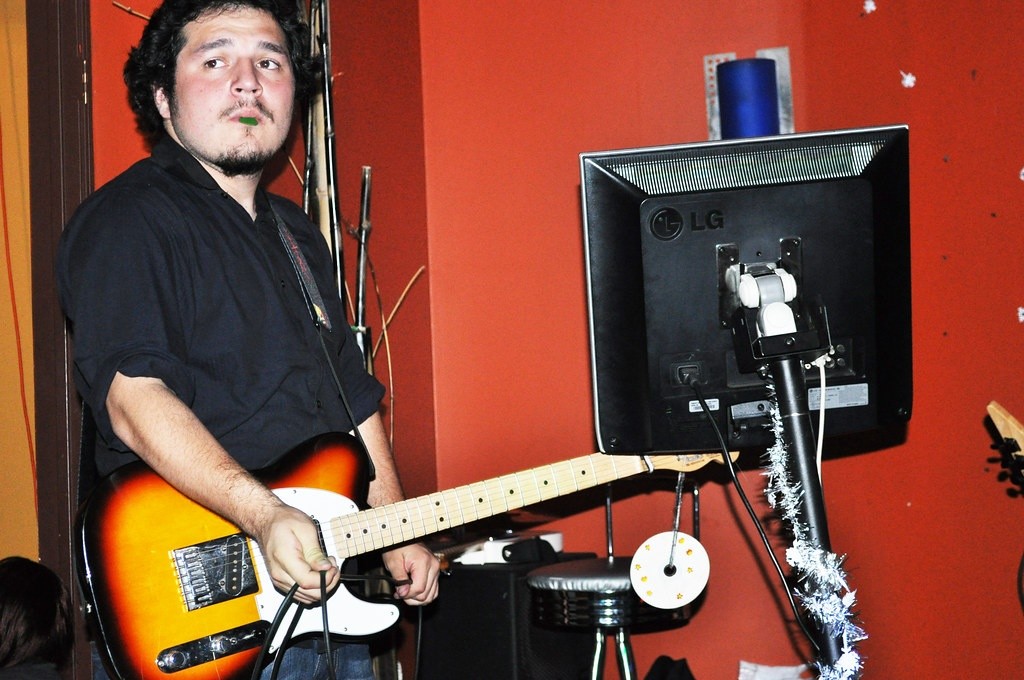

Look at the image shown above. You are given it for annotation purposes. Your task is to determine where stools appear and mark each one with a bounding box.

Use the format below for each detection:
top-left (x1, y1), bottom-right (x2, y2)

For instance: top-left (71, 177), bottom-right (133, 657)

top-left (525, 556), bottom-right (636, 680)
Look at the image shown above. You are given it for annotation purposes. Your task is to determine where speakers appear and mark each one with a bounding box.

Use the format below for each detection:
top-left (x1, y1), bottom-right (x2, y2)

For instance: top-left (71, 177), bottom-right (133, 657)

top-left (411, 551), bottom-right (607, 680)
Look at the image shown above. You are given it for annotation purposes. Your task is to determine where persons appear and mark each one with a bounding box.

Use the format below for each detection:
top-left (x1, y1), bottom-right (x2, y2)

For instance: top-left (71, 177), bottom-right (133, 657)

top-left (55, 0), bottom-right (440, 680)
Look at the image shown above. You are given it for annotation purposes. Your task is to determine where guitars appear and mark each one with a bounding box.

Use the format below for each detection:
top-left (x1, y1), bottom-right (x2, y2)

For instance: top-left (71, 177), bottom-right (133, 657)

top-left (71, 435), bottom-right (741, 679)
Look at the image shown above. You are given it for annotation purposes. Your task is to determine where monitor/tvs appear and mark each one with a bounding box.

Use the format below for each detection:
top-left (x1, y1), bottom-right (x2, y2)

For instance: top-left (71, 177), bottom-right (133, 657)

top-left (577, 122), bottom-right (910, 456)
